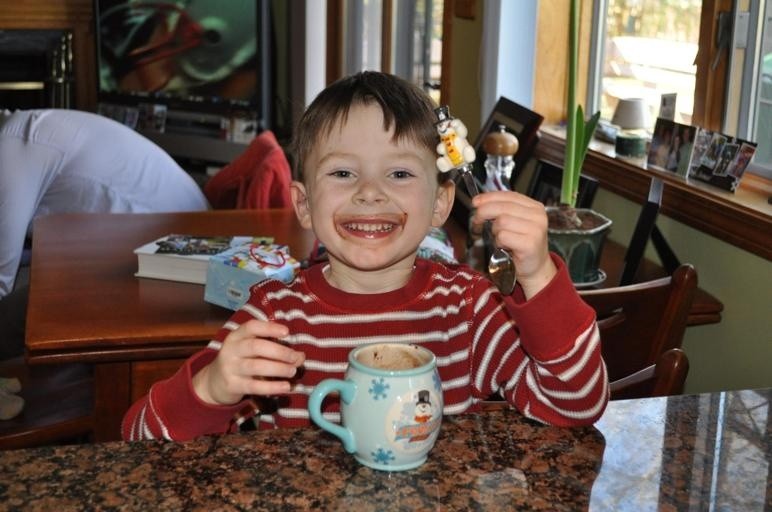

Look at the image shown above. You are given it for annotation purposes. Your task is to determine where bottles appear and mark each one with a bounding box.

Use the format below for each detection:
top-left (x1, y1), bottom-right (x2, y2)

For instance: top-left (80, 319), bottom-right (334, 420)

top-left (462, 124), bottom-right (520, 275)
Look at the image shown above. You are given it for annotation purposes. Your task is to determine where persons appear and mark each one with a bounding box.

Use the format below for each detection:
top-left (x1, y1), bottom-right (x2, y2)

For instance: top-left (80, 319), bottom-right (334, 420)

top-left (664, 134), bottom-right (682, 173)
top-left (122, 69), bottom-right (613, 444)
top-left (1, 107), bottom-right (218, 364)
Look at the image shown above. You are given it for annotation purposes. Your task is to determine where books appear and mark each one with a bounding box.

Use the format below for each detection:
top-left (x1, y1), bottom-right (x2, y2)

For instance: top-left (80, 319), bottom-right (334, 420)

top-left (131, 232), bottom-right (276, 286)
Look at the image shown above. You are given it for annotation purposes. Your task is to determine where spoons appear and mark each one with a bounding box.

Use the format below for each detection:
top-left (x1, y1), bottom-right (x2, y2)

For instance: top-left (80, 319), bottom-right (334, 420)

top-left (433, 104), bottom-right (518, 297)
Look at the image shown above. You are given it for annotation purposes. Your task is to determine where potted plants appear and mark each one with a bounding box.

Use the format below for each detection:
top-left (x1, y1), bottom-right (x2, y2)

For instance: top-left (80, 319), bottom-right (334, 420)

top-left (542, 0), bottom-right (613, 290)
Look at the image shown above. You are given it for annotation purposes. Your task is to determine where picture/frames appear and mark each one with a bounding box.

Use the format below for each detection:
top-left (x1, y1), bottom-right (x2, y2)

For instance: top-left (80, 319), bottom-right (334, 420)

top-left (618, 178), bottom-right (682, 285)
top-left (471, 96), bottom-right (599, 209)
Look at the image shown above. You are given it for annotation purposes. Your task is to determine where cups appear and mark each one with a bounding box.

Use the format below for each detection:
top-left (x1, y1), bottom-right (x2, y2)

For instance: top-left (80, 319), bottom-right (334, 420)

top-left (308, 342), bottom-right (444, 472)
